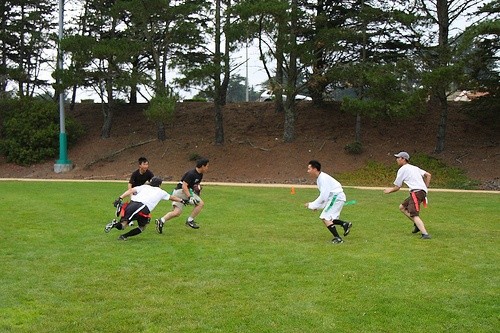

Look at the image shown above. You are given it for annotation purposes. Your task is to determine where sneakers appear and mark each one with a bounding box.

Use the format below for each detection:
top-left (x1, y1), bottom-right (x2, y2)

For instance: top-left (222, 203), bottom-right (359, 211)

top-left (155, 219), bottom-right (163, 233)
top-left (185, 220), bottom-right (199, 229)
top-left (343, 222), bottom-right (352, 236)
top-left (332, 237), bottom-right (343, 244)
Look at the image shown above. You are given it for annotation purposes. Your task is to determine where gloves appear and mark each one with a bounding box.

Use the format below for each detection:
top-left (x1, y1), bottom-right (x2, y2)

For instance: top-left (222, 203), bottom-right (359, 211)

top-left (193, 184), bottom-right (201, 195)
top-left (113, 198), bottom-right (123, 207)
top-left (181, 199), bottom-right (189, 206)
top-left (188, 197), bottom-right (198, 206)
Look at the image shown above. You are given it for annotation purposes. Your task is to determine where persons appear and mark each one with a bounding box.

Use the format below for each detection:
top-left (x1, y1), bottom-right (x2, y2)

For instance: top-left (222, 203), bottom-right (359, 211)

top-left (114, 157), bottom-right (155, 226)
top-left (155, 159), bottom-right (210, 234)
top-left (303, 160), bottom-right (352, 244)
top-left (384, 152), bottom-right (431, 240)
top-left (105, 177), bottom-right (190, 241)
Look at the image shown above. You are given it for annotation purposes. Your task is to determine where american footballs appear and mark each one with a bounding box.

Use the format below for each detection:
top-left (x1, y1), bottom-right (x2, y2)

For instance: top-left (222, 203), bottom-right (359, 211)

top-left (192, 184), bottom-right (203, 193)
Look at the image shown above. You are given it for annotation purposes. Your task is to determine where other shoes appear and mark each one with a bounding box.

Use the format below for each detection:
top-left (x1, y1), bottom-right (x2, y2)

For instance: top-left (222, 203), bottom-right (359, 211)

top-left (412, 226), bottom-right (420, 233)
top-left (115, 203), bottom-right (122, 217)
top-left (104, 218), bottom-right (117, 233)
top-left (120, 236), bottom-right (127, 241)
top-left (421, 234), bottom-right (431, 239)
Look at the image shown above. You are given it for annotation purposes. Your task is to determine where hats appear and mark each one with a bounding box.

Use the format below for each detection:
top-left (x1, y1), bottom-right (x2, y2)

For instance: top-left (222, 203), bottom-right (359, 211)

top-left (394, 152), bottom-right (409, 160)
